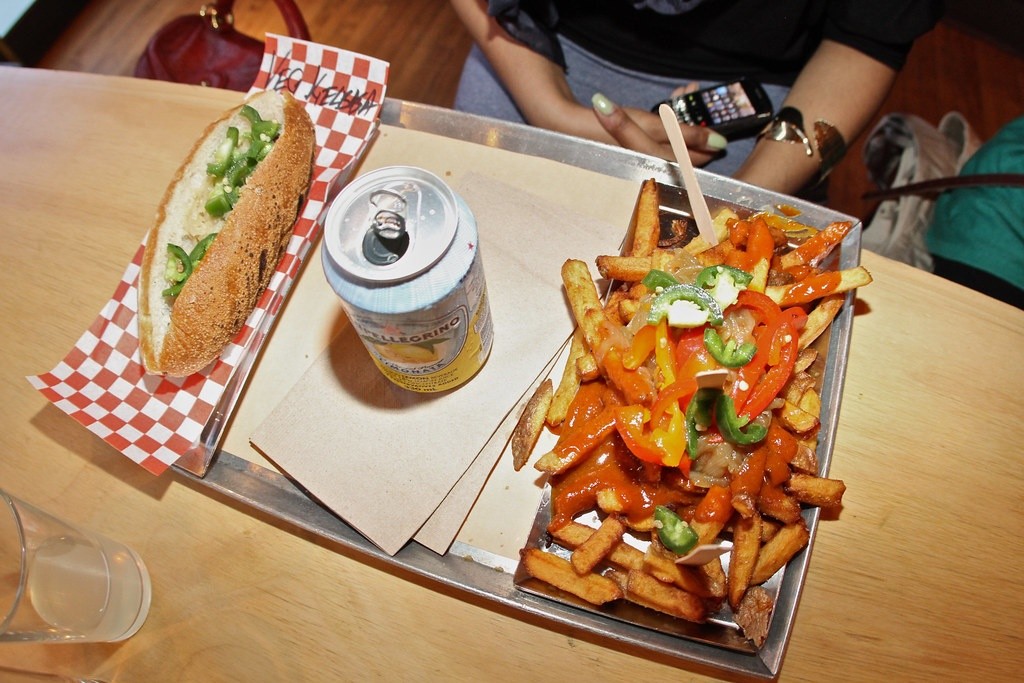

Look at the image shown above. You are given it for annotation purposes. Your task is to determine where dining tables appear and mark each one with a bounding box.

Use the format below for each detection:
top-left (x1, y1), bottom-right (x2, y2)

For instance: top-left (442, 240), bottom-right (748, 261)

top-left (0, 67), bottom-right (1024, 683)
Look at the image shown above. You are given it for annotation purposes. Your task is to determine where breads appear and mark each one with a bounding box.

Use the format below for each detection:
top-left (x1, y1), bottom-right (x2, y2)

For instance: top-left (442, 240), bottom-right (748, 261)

top-left (135, 89), bottom-right (316, 377)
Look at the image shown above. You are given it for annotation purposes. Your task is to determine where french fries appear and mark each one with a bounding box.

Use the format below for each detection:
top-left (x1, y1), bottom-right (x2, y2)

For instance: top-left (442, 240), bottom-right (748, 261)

top-left (510, 179), bottom-right (869, 649)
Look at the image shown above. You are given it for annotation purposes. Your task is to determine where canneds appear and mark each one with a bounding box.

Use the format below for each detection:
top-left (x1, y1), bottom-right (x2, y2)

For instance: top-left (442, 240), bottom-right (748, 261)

top-left (319, 165), bottom-right (496, 394)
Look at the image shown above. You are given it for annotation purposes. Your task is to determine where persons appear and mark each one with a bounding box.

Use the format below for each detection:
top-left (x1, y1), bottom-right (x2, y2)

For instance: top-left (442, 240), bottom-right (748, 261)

top-left (448, 1), bottom-right (944, 209)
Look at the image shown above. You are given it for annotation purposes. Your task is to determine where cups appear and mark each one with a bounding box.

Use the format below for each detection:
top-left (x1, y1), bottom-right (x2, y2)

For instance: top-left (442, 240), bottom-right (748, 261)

top-left (0, 483), bottom-right (153, 647)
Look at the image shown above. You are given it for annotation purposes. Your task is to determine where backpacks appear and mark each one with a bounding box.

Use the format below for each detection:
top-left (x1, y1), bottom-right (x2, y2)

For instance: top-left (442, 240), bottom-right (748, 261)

top-left (131, 0), bottom-right (313, 93)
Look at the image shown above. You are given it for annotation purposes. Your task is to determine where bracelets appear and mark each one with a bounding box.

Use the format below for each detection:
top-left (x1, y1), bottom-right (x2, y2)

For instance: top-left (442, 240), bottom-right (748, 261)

top-left (753, 106), bottom-right (848, 191)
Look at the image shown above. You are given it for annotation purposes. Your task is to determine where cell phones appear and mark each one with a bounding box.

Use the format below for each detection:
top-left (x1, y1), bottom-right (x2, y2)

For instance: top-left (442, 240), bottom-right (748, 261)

top-left (650, 79), bottom-right (775, 142)
